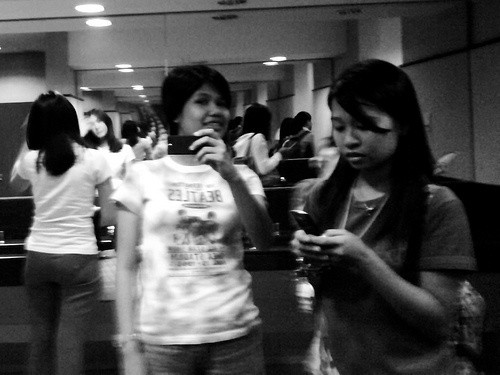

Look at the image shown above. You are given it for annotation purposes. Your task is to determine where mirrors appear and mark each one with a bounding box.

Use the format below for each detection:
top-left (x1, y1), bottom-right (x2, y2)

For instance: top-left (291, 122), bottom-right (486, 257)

top-left (74, 57), bottom-right (335, 199)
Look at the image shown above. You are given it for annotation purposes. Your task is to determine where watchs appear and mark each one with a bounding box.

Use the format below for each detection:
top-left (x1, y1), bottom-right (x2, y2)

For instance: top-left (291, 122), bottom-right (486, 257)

top-left (112, 334), bottom-right (140, 346)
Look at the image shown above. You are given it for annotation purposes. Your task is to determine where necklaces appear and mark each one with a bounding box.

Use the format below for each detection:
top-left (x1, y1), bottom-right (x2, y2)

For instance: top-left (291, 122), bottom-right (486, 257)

top-left (357, 178), bottom-right (383, 211)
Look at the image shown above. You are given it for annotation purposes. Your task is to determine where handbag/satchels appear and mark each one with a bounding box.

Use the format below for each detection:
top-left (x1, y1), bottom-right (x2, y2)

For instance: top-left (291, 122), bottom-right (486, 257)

top-left (233, 134), bottom-right (260, 172)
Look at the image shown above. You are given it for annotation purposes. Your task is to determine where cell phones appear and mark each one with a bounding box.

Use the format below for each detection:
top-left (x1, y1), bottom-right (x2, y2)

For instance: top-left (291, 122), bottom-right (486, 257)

top-left (167, 135), bottom-right (213, 155)
top-left (284, 141), bottom-right (298, 150)
top-left (289, 209), bottom-right (316, 241)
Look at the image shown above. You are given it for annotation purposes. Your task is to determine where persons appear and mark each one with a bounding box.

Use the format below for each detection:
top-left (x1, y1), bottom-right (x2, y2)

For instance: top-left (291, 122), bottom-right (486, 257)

top-left (290, 58), bottom-right (476, 374)
top-left (10, 91), bottom-right (114, 375)
top-left (271, 112), bottom-right (314, 174)
top-left (233, 103), bottom-right (291, 176)
top-left (115, 65), bottom-right (273, 375)
top-left (79, 108), bottom-right (152, 179)
